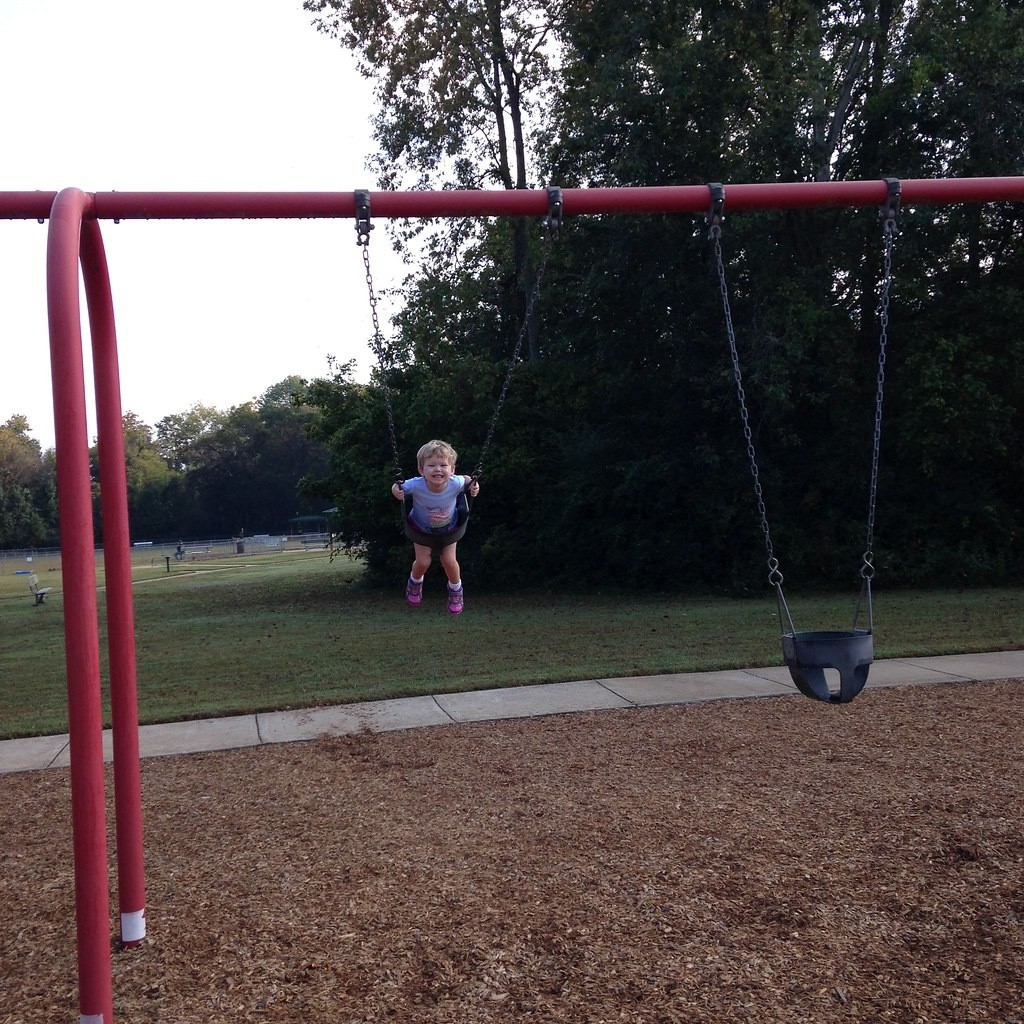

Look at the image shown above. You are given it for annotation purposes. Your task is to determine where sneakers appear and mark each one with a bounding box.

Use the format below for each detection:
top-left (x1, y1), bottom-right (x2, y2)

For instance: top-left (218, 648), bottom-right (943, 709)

top-left (447, 583), bottom-right (464, 615)
top-left (406, 577), bottom-right (423, 607)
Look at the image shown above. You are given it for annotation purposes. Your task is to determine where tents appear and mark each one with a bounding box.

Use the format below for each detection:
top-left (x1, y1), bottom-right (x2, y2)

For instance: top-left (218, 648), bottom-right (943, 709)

top-left (289, 514), bottom-right (326, 540)
top-left (321, 507), bottom-right (341, 545)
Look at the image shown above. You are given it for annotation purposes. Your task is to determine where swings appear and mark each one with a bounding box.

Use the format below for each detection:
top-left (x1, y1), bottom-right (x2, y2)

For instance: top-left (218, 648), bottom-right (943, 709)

top-left (706, 209), bottom-right (901, 704)
top-left (356, 219), bottom-right (561, 547)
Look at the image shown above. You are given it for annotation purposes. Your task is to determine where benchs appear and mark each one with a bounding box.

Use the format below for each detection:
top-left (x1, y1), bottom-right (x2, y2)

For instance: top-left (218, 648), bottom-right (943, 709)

top-left (27, 574), bottom-right (53, 607)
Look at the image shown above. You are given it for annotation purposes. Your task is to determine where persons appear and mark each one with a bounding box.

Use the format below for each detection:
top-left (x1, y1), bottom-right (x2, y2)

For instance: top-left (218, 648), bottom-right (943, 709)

top-left (174, 536), bottom-right (186, 561)
top-left (392, 440), bottom-right (480, 614)
top-left (239, 527), bottom-right (245, 540)
